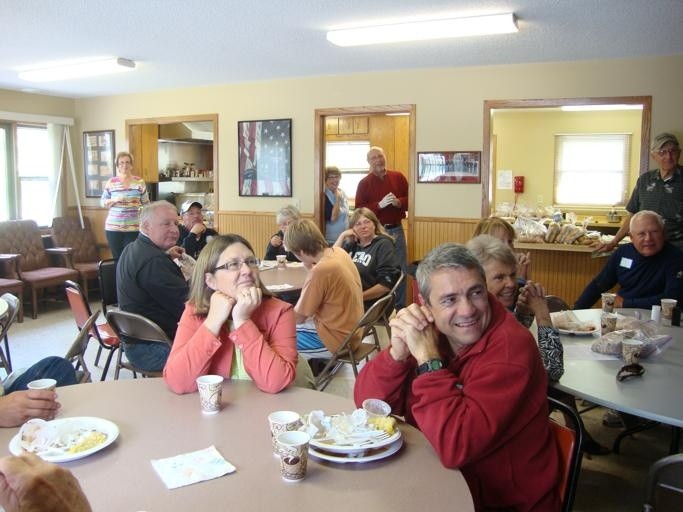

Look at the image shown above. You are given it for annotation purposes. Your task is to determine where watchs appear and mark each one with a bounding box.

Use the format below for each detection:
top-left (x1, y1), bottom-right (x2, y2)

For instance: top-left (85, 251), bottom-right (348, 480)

top-left (408, 356), bottom-right (448, 377)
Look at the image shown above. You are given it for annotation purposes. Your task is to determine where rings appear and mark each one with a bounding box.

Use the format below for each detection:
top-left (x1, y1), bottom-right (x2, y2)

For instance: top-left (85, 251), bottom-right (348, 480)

top-left (243, 292), bottom-right (250, 296)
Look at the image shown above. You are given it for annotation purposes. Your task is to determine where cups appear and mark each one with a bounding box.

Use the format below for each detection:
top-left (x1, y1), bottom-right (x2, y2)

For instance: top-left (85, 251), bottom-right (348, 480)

top-left (276, 430), bottom-right (309, 483)
top-left (361, 399), bottom-right (392, 418)
top-left (267, 411), bottom-right (301, 459)
top-left (27, 378), bottom-right (57, 421)
top-left (196, 375), bottom-right (225, 416)
top-left (276, 255), bottom-right (287, 270)
top-left (600, 292), bottom-right (677, 365)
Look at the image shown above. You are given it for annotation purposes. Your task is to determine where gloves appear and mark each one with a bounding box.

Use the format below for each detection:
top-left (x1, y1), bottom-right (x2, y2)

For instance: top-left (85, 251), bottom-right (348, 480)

top-left (378, 193), bottom-right (393, 208)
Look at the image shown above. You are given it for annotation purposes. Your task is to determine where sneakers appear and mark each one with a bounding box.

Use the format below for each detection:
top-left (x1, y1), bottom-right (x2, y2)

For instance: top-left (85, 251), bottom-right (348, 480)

top-left (602, 410), bottom-right (640, 426)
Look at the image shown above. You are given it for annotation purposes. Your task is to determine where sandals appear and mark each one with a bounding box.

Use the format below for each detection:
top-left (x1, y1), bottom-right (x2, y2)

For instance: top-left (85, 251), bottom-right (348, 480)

top-left (574, 430), bottom-right (611, 456)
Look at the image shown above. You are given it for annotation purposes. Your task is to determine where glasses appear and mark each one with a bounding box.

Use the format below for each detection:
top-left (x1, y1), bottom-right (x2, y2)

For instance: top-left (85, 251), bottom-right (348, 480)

top-left (615, 364), bottom-right (644, 382)
top-left (118, 161), bottom-right (131, 166)
top-left (656, 148), bottom-right (676, 155)
top-left (327, 176), bottom-right (340, 180)
top-left (211, 257), bottom-right (260, 271)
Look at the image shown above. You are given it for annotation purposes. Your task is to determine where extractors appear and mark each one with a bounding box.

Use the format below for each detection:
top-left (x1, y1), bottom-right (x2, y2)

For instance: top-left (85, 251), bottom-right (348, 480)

top-left (158, 122), bottom-right (213, 145)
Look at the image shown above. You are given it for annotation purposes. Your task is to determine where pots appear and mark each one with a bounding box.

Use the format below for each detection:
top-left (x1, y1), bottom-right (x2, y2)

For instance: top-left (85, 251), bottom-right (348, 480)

top-left (607, 215), bottom-right (623, 223)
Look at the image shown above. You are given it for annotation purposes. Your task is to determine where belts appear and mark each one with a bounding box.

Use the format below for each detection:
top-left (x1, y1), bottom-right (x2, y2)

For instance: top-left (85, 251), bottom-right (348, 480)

top-left (385, 223), bottom-right (399, 229)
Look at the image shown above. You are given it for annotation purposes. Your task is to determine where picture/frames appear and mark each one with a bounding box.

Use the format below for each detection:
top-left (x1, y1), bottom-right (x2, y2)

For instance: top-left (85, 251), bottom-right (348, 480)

top-left (237, 118), bottom-right (292, 198)
top-left (82, 129), bottom-right (117, 198)
top-left (416, 150), bottom-right (482, 184)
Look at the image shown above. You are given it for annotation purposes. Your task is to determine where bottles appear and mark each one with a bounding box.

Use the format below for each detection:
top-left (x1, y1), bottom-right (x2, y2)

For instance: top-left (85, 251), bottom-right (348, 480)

top-left (167, 164), bottom-right (213, 178)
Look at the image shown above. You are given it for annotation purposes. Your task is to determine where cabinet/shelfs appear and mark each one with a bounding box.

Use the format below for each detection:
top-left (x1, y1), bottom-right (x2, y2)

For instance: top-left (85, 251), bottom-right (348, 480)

top-left (128, 125), bottom-right (159, 184)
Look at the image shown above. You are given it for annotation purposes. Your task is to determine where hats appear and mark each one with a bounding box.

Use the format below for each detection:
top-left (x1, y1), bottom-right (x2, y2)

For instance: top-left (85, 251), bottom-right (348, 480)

top-left (180, 199), bottom-right (202, 214)
top-left (650, 133), bottom-right (678, 151)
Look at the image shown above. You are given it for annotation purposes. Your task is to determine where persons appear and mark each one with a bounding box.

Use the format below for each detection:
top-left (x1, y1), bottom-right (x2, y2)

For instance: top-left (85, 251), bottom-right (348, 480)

top-left (0, 445), bottom-right (96, 511)
top-left (347, 241), bottom-right (569, 512)
top-left (588, 130), bottom-right (682, 255)
top-left (325, 165), bottom-right (353, 248)
top-left (264, 207), bottom-right (301, 305)
top-left (284, 217), bottom-right (364, 362)
top-left (0, 353), bottom-right (80, 428)
top-left (98, 151), bottom-right (151, 265)
top-left (459, 229), bottom-right (565, 384)
top-left (163, 234), bottom-right (298, 394)
top-left (570, 209), bottom-right (682, 429)
top-left (332, 208), bottom-right (401, 314)
top-left (470, 214), bottom-right (611, 458)
top-left (115, 201), bottom-right (190, 372)
top-left (354, 146), bottom-right (409, 311)
top-left (175, 201), bottom-right (219, 262)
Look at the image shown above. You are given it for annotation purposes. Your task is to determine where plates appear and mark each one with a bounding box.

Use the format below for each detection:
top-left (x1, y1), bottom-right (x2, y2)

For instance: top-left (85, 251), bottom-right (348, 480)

top-left (306, 415), bottom-right (404, 465)
top-left (8, 416), bottom-right (119, 463)
top-left (557, 323), bottom-right (600, 336)
